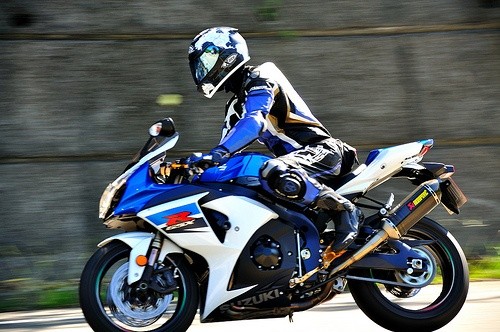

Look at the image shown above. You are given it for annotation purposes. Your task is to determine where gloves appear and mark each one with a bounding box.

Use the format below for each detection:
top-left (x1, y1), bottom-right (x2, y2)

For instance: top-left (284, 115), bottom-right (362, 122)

top-left (179, 152), bottom-right (213, 176)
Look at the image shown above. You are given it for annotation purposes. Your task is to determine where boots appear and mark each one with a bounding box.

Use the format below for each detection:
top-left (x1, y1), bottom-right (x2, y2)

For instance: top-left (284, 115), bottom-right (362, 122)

top-left (314, 185), bottom-right (365, 253)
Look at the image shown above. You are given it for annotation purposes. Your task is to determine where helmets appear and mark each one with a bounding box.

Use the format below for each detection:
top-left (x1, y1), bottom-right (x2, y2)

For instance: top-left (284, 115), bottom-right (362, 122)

top-left (188, 25), bottom-right (249, 98)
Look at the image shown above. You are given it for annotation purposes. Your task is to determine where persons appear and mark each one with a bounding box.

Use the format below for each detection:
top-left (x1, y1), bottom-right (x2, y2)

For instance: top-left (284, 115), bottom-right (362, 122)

top-left (182, 26), bottom-right (365, 252)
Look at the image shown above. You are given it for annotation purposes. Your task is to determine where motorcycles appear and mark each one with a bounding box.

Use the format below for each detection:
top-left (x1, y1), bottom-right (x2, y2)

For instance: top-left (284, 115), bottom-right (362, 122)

top-left (77, 118), bottom-right (470, 332)
